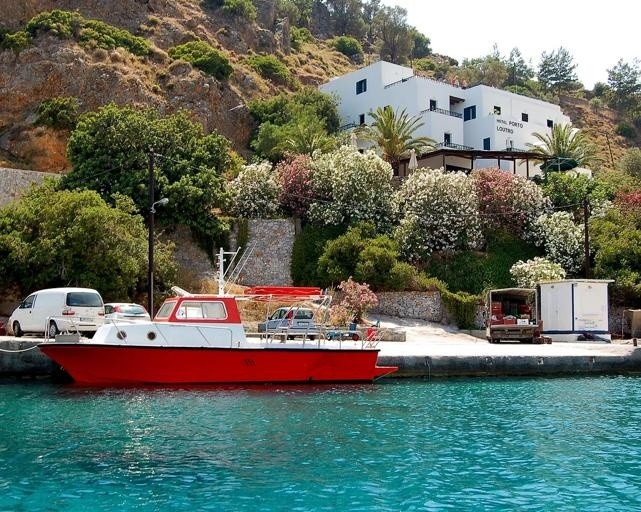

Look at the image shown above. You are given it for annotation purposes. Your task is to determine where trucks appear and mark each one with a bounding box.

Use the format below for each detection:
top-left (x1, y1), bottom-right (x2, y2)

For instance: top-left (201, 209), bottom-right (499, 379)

top-left (482, 288), bottom-right (539, 344)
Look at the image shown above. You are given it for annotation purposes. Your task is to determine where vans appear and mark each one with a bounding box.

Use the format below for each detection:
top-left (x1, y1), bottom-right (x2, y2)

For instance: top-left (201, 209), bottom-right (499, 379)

top-left (7, 287), bottom-right (105, 338)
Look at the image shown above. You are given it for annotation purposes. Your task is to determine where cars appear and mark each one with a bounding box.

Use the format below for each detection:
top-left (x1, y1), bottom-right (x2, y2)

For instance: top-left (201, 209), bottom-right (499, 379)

top-left (104, 303), bottom-right (151, 322)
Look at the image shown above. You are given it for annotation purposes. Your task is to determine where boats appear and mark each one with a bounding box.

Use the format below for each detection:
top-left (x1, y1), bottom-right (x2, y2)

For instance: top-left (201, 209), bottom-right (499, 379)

top-left (35, 295), bottom-right (399, 385)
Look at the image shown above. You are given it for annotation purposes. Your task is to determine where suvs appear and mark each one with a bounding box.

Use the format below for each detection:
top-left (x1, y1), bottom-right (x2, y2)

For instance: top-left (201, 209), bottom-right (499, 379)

top-left (258, 306), bottom-right (318, 340)
